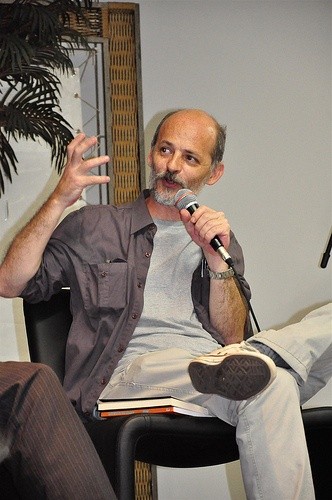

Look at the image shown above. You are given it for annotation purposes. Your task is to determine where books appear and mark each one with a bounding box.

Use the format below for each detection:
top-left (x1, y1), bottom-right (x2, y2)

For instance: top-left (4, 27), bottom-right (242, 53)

top-left (98, 394), bottom-right (211, 420)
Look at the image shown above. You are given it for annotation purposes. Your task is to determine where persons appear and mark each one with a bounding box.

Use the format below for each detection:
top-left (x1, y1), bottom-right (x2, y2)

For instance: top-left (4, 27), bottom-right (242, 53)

top-left (0, 360), bottom-right (116, 500)
top-left (0, 109), bottom-right (332, 500)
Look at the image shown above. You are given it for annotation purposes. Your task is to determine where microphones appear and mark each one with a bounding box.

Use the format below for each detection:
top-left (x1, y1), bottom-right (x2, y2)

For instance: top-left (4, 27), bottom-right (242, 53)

top-left (174, 188), bottom-right (234, 267)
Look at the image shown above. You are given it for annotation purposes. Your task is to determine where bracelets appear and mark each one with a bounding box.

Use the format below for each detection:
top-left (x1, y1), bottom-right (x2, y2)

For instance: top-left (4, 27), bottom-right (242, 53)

top-left (209, 269), bottom-right (239, 282)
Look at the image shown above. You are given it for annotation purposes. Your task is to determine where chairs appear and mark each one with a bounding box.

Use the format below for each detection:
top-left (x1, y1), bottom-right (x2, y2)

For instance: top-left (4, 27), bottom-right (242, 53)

top-left (24, 289), bottom-right (332, 500)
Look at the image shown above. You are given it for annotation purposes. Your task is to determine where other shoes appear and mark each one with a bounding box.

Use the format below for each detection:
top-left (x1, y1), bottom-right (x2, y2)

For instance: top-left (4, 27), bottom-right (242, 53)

top-left (189, 341), bottom-right (277, 402)
